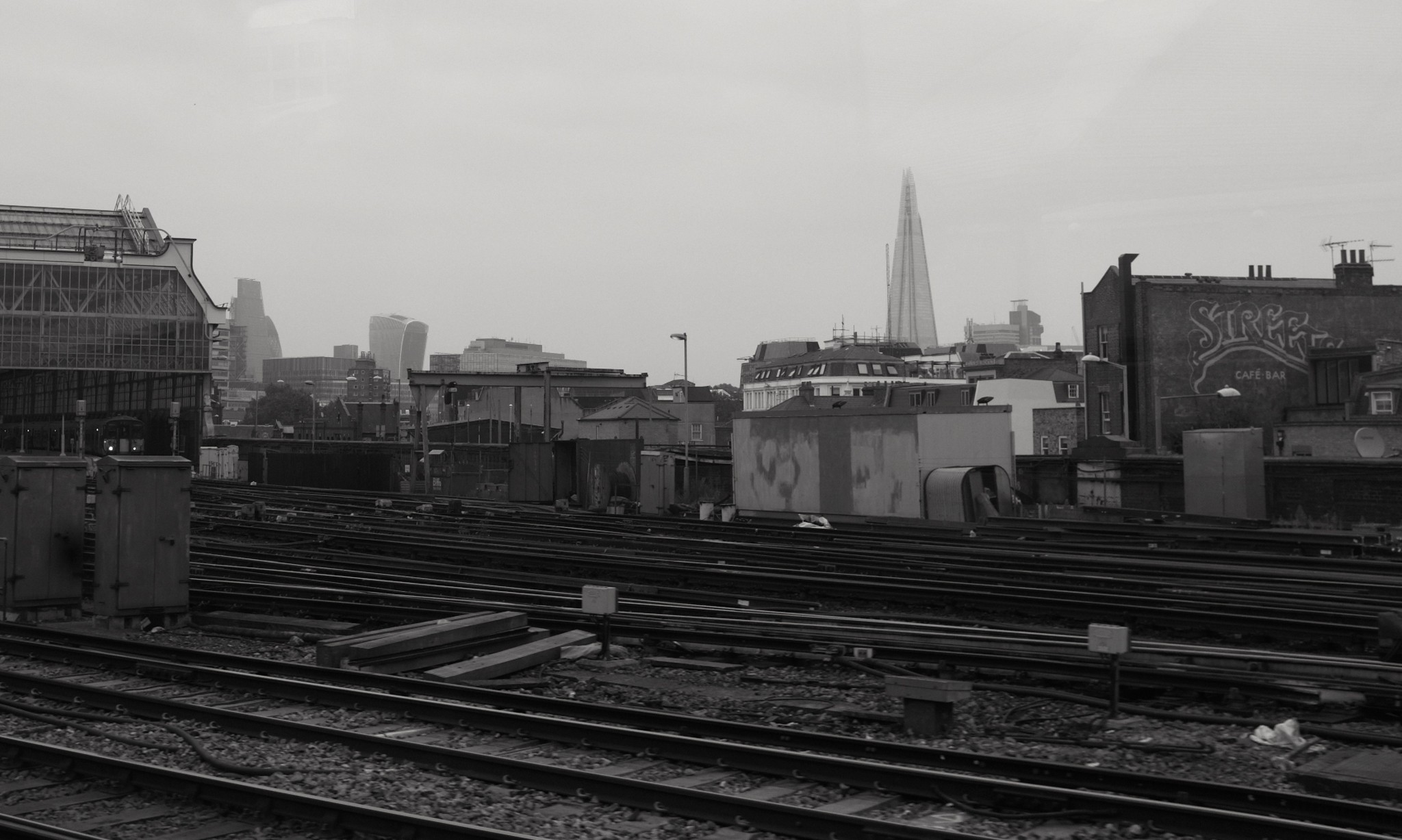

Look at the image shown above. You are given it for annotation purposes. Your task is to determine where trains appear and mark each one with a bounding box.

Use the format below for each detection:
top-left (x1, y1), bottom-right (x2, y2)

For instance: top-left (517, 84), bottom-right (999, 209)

top-left (0, 413), bottom-right (145, 455)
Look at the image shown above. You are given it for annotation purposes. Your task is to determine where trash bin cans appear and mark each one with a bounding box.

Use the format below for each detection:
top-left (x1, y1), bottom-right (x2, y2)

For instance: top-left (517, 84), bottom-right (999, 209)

top-left (720, 503), bottom-right (737, 522)
top-left (700, 501), bottom-right (714, 521)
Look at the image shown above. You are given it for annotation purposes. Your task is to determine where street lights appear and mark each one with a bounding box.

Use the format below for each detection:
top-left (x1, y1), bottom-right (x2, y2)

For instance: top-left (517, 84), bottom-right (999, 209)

top-left (373, 376), bottom-right (401, 442)
top-left (670, 333), bottom-right (691, 505)
top-left (255, 379), bottom-right (284, 438)
top-left (304, 380), bottom-right (315, 450)
top-left (1080, 351), bottom-right (1129, 440)
